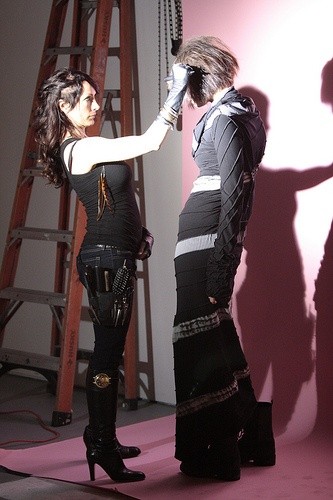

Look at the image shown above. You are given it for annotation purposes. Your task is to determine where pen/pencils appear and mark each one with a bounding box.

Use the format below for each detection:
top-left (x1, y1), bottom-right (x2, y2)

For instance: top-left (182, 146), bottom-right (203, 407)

top-left (163, 70), bottom-right (194, 81)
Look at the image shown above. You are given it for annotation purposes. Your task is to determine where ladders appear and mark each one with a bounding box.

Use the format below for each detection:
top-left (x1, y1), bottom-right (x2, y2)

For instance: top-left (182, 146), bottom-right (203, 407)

top-left (0, 0), bottom-right (134, 428)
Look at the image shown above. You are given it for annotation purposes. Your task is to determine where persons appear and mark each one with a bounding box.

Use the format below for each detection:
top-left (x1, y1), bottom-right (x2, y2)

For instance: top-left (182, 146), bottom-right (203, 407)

top-left (172, 34), bottom-right (277, 481)
top-left (31, 60), bottom-right (194, 482)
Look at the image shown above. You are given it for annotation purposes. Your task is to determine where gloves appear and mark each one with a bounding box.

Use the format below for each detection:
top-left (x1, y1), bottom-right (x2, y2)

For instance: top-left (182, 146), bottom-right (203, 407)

top-left (158, 63), bottom-right (194, 127)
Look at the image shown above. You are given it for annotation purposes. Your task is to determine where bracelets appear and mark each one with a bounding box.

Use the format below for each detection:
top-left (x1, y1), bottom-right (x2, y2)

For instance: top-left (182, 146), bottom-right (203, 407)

top-left (156, 106), bottom-right (176, 130)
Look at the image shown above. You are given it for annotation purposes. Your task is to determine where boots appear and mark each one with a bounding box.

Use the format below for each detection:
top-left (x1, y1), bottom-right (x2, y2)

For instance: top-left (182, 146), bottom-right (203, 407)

top-left (238, 402), bottom-right (275, 467)
top-left (181, 431), bottom-right (241, 481)
top-left (83, 369), bottom-right (146, 483)
